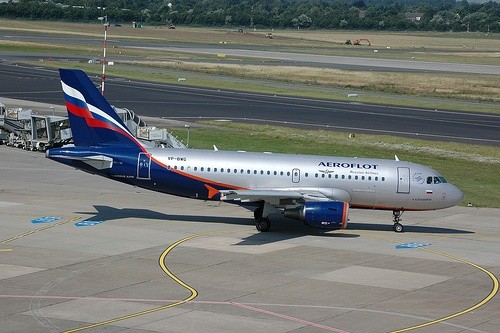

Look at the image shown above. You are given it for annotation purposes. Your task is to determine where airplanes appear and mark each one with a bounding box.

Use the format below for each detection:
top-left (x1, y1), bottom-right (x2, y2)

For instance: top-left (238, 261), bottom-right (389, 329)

top-left (46, 68), bottom-right (464, 233)
